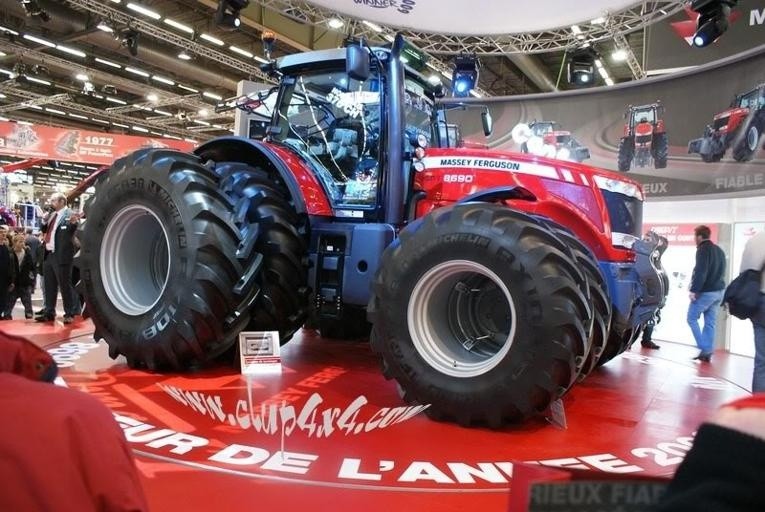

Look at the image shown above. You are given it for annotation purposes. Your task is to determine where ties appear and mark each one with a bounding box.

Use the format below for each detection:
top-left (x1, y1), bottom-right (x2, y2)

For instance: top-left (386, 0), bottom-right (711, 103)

top-left (45, 213), bottom-right (57, 242)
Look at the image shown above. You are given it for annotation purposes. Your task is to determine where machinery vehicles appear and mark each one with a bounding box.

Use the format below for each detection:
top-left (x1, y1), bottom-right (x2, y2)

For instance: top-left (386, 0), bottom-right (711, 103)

top-left (617, 99), bottom-right (668, 172)
top-left (686, 82), bottom-right (765, 163)
top-left (519, 119), bottom-right (591, 163)
top-left (64, 30), bottom-right (670, 429)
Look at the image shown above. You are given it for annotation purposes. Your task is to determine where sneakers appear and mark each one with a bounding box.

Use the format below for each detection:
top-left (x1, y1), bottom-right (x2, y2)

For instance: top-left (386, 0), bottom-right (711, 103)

top-left (26, 312), bottom-right (33, 319)
top-left (2, 316), bottom-right (12, 320)
top-left (694, 351), bottom-right (711, 362)
top-left (643, 341), bottom-right (659, 349)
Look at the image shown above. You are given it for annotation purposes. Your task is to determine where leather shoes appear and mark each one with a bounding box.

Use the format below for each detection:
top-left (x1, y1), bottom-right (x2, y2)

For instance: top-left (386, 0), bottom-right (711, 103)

top-left (63, 316), bottom-right (73, 323)
top-left (34, 309), bottom-right (56, 321)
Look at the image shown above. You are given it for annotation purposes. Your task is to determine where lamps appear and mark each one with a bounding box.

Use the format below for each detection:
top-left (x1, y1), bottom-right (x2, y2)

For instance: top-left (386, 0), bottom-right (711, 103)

top-left (565, 26), bottom-right (615, 90)
top-left (1, 0), bottom-right (252, 144)
top-left (452, 55), bottom-right (481, 94)
top-left (689, 1), bottom-right (732, 48)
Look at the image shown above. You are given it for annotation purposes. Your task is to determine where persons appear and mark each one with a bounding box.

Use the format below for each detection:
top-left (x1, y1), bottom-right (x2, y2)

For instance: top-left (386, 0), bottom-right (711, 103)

top-left (33, 192), bottom-right (82, 324)
top-left (0, 223), bottom-right (91, 324)
top-left (737, 229), bottom-right (765, 396)
top-left (686, 224), bottom-right (728, 363)
top-left (642, 230), bottom-right (662, 350)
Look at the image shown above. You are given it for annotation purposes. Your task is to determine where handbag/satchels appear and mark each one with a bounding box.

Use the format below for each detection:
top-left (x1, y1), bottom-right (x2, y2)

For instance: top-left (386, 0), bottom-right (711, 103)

top-left (720, 269), bottom-right (761, 319)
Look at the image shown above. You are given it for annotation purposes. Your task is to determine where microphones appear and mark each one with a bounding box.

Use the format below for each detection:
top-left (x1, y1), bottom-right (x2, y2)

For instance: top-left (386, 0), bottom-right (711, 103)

top-left (47, 208), bottom-right (54, 214)
